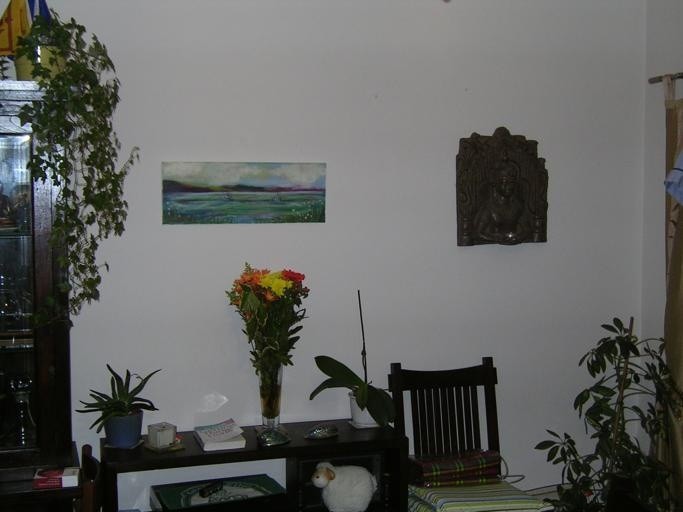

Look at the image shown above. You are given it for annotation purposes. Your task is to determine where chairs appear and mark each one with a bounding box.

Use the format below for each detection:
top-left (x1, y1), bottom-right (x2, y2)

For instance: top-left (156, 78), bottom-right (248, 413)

top-left (381, 356), bottom-right (543, 511)
top-left (77, 443), bottom-right (103, 512)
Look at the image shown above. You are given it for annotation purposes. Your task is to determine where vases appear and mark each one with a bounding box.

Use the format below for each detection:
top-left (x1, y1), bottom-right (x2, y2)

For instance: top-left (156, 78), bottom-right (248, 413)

top-left (254, 363), bottom-right (290, 447)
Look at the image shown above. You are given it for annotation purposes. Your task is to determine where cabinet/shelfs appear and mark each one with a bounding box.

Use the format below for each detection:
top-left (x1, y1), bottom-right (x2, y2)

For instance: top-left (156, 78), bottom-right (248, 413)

top-left (0, 81), bottom-right (84, 510)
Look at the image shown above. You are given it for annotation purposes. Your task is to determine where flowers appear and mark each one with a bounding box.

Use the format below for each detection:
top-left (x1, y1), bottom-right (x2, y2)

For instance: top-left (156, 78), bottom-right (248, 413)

top-left (224, 262), bottom-right (311, 414)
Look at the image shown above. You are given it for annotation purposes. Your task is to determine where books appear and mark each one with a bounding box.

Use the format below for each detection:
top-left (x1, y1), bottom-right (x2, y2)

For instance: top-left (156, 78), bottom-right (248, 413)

top-left (193, 417), bottom-right (247, 452)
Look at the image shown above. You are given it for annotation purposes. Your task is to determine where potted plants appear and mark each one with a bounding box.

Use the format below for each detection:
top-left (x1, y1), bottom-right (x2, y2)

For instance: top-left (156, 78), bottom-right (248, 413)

top-left (308, 354), bottom-right (394, 429)
top-left (10, 10), bottom-right (139, 320)
top-left (73, 363), bottom-right (164, 449)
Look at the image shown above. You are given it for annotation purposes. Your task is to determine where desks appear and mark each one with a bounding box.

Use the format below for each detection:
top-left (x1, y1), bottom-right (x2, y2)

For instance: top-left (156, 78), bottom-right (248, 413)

top-left (101, 418), bottom-right (409, 510)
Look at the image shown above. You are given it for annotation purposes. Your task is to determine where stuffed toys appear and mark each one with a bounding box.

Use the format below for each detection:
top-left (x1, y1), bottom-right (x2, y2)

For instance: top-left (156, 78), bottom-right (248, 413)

top-left (310, 461), bottom-right (378, 512)
